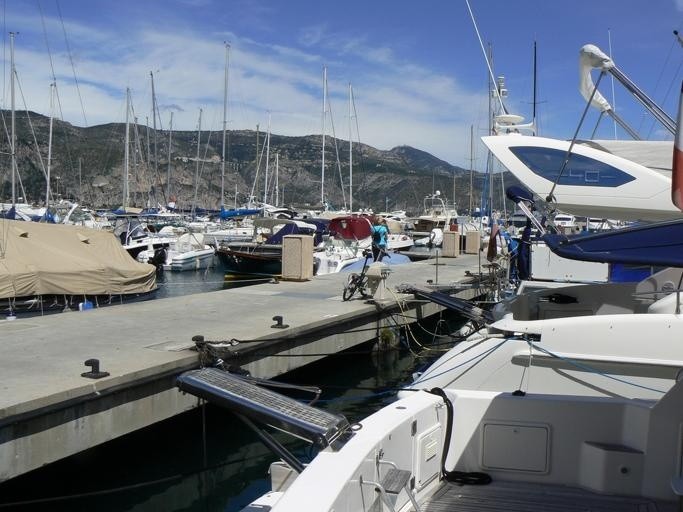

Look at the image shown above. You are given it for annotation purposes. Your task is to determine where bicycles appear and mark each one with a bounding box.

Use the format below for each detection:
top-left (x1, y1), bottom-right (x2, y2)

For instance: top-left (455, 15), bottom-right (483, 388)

top-left (340, 242), bottom-right (392, 302)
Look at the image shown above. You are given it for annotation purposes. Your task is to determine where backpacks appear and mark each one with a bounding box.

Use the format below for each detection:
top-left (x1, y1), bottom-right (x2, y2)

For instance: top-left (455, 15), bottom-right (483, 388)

top-left (373, 225), bottom-right (383, 242)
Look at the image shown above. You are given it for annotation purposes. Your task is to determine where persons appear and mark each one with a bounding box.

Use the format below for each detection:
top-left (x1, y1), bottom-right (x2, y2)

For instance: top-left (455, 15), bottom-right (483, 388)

top-left (370, 214), bottom-right (389, 262)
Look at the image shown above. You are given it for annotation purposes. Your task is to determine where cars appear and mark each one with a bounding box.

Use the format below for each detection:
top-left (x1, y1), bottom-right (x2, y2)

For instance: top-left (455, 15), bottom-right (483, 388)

top-left (552, 214), bottom-right (627, 233)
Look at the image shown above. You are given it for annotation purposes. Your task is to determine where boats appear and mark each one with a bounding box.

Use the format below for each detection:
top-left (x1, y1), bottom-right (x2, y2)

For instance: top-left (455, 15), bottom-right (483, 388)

top-left (178, 266), bottom-right (683, 511)
top-left (463, 0), bottom-right (683, 285)
